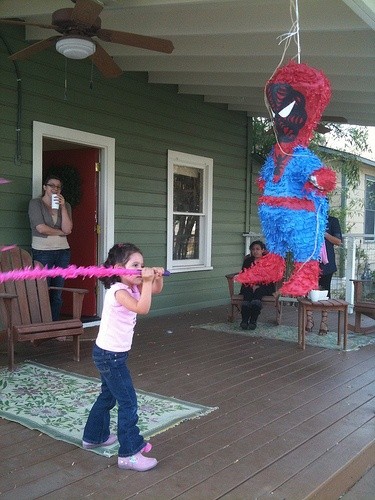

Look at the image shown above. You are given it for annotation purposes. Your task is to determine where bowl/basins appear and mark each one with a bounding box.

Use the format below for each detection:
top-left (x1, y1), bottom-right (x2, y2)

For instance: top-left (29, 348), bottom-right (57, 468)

top-left (310, 290), bottom-right (328, 297)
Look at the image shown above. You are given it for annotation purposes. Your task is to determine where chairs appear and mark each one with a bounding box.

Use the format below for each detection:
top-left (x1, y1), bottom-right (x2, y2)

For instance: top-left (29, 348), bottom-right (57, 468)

top-left (351, 278), bottom-right (375, 333)
top-left (225, 269), bottom-right (283, 324)
top-left (0, 245), bottom-right (89, 370)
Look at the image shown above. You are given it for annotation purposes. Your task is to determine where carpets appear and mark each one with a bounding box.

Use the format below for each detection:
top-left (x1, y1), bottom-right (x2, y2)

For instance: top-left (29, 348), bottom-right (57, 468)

top-left (191, 319), bottom-right (375, 351)
top-left (0, 360), bottom-right (221, 458)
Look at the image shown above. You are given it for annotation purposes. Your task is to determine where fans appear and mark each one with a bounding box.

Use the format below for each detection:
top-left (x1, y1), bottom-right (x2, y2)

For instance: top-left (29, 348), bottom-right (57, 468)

top-left (266, 115), bottom-right (348, 135)
top-left (0, 0), bottom-right (175, 81)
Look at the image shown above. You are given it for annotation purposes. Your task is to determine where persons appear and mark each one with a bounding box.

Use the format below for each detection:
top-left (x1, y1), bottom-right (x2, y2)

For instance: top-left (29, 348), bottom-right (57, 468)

top-left (82, 243), bottom-right (165, 472)
top-left (239, 240), bottom-right (276, 330)
top-left (28, 175), bottom-right (73, 342)
top-left (306, 198), bottom-right (342, 336)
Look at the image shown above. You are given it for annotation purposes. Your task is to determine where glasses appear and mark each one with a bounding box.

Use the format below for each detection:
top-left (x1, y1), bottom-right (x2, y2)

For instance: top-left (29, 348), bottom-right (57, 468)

top-left (46, 183), bottom-right (62, 190)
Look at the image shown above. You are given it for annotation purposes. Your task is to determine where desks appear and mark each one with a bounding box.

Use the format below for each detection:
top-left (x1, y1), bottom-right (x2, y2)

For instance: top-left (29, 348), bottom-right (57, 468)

top-left (296, 297), bottom-right (349, 351)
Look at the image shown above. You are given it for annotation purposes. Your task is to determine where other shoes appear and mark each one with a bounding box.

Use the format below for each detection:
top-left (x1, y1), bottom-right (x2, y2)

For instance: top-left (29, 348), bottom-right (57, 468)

top-left (117, 452), bottom-right (157, 472)
top-left (83, 435), bottom-right (118, 449)
top-left (249, 319), bottom-right (257, 329)
top-left (240, 318), bottom-right (248, 328)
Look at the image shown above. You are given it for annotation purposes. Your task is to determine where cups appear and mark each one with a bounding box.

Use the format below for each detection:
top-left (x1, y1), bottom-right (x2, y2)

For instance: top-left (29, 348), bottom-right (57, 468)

top-left (52, 193), bottom-right (60, 210)
top-left (310, 290), bottom-right (318, 302)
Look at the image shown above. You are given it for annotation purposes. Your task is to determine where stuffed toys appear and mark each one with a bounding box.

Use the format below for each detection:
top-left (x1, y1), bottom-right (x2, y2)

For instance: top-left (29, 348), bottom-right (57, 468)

top-left (232, 62), bottom-right (337, 296)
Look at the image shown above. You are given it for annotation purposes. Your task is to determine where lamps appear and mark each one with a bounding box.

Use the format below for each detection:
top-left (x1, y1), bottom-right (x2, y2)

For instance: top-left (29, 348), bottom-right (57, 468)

top-left (55, 38), bottom-right (97, 60)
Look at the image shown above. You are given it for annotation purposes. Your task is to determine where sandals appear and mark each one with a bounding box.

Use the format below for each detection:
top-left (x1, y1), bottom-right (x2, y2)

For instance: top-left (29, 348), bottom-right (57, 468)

top-left (306, 310), bottom-right (314, 331)
top-left (319, 310), bottom-right (328, 335)
